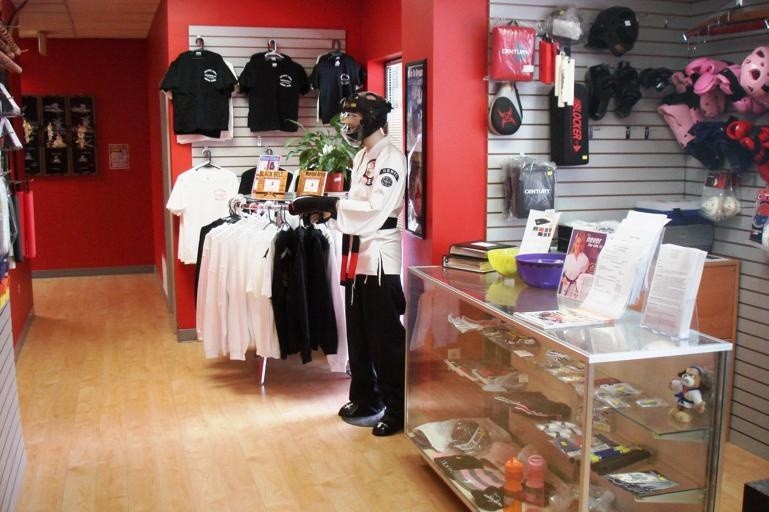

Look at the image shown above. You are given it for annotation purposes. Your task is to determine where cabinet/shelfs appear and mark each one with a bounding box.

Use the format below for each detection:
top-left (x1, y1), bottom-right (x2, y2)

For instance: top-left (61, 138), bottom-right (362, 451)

top-left (405, 265), bottom-right (732, 512)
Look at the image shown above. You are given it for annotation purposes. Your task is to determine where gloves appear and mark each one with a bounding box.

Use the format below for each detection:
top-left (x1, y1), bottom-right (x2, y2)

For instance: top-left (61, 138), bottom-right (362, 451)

top-left (288, 195), bottom-right (340, 216)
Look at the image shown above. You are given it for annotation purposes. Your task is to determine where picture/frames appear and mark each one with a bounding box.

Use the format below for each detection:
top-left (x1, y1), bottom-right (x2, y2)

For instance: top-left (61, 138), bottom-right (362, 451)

top-left (257, 170), bottom-right (328, 195)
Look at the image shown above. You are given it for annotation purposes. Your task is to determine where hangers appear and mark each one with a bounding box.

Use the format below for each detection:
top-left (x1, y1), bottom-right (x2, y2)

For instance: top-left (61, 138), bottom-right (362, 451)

top-left (220, 196), bottom-right (308, 233)
top-left (195, 149), bottom-right (220, 172)
top-left (185, 35), bottom-right (343, 63)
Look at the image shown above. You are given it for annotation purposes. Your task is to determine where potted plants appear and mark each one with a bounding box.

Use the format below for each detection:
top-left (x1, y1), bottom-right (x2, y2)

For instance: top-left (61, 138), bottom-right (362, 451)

top-left (283, 118), bottom-right (365, 192)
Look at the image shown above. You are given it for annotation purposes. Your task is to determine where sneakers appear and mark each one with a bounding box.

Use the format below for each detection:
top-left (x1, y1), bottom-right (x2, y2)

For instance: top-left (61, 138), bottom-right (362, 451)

top-left (338, 401), bottom-right (402, 436)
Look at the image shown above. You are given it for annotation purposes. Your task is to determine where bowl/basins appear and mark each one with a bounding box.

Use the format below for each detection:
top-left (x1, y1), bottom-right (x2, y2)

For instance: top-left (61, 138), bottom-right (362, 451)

top-left (515, 253), bottom-right (566, 289)
top-left (487, 248), bottom-right (518, 278)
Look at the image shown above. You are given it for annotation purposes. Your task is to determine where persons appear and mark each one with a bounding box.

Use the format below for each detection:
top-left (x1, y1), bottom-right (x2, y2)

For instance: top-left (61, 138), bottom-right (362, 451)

top-left (561, 231), bottom-right (589, 300)
top-left (289, 92), bottom-right (408, 436)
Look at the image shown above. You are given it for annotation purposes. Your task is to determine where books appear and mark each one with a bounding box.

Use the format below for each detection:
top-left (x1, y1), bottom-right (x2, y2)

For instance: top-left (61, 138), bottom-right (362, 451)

top-left (513, 308), bottom-right (616, 329)
top-left (442, 241), bottom-right (517, 273)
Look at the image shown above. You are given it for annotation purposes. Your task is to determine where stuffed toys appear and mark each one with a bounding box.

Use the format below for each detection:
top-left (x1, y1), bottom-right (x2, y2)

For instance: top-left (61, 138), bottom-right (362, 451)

top-left (668, 364), bottom-right (711, 423)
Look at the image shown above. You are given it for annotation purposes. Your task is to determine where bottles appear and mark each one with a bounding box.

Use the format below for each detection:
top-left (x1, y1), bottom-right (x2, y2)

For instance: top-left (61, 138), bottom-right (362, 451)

top-left (525, 454), bottom-right (546, 505)
top-left (502, 453), bottom-right (524, 511)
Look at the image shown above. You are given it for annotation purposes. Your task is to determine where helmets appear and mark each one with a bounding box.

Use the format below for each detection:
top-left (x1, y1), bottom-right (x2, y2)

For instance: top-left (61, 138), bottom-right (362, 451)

top-left (339, 91), bottom-right (392, 142)
top-left (589, 6), bottom-right (638, 59)
